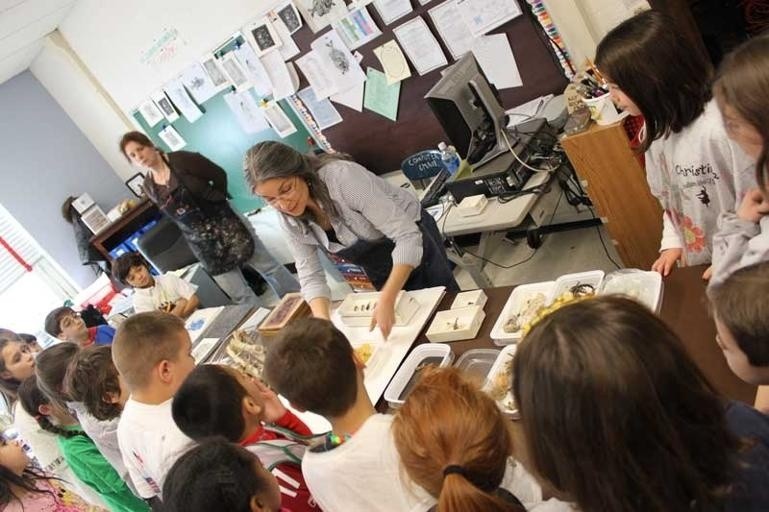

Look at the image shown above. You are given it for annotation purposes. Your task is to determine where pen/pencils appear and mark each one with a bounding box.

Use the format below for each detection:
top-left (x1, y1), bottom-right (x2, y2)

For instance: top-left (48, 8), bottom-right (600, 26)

top-left (569, 54), bottom-right (608, 100)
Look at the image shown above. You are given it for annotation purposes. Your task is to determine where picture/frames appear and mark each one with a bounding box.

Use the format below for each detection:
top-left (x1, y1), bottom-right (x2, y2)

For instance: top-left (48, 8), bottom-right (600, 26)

top-left (125, 171), bottom-right (146, 201)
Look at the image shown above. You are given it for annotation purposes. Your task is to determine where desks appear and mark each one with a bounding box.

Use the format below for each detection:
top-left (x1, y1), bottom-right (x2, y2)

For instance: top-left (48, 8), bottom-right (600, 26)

top-left (419, 97), bottom-right (565, 289)
top-left (86, 193), bottom-right (158, 269)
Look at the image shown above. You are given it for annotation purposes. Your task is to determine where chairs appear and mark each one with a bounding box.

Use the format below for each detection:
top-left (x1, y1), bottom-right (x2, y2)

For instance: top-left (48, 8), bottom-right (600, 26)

top-left (400, 148), bottom-right (445, 189)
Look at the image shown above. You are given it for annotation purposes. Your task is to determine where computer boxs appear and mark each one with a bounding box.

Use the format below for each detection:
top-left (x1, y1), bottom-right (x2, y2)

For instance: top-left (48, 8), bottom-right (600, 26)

top-left (444, 118), bottom-right (559, 196)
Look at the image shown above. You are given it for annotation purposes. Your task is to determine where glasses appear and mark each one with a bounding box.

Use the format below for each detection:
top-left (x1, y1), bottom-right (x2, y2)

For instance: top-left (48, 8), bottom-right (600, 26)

top-left (61, 312), bottom-right (81, 334)
top-left (259, 176), bottom-right (297, 207)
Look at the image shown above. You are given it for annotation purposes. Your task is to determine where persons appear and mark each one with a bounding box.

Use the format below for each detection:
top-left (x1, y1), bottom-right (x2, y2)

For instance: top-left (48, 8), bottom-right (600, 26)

top-left (64, 347), bottom-right (129, 422)
top-left (702, 32), bottom-right (769, 290)
top-left (118, 132), bottom-right (302, 306)
top-left (20, 374), bottom-right (150, 511)
top-left (242, 141), bottom-right (459, 341)
top-left (61, 197), bottom-right (112, 276)
top-left (392, 367), bottom-right (530, 511)
top-left (111, 312), bottom-right (196, 511)
top-left (512, 299), bottom-right (768, 511)
top-left (33, 341), bottom-right (150, 511)
top-left (593, 10), bottom-right (755, 277)
top-left (0, 434), bottom-right (106, 512)
top-left (162, 440), bottom-right (284, 511)
top-left (703, 263), bottom-right (769, 415)
top-left (171, 364), bottom-right (321, 512)
top-left (117, 251), bottom-right (199, 318)
top-left (45, 306), bottom-right (119, 349)
top-left (0, 329), bottom-right (108, 512)
top-left (264, 317), bottom-right (581, 512)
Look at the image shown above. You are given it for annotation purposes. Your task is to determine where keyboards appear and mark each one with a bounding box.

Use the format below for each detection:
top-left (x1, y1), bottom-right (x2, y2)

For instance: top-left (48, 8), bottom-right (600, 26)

top-left (418, 167), bottom-right (451, 208)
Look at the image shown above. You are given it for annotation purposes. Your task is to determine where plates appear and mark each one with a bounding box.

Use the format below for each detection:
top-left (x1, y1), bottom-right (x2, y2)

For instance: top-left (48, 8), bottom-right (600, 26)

top-left (604, 270), bottom-right (663, 315)
top-left (210, 325), bottom-right (258, 368)
top-left (491, 281), bottom-right (558, 345)
top-left (452, 343), bottom-right (500, 392)
top-left (384, 343), bottom-right (454, 410)
top-left (481, 344), bottom-right (537, 422)
top-left (548, 270), bottom-right (604, 308)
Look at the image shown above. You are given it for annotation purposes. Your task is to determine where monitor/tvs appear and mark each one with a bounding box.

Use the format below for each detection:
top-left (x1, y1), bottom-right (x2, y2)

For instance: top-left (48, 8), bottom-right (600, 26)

top-left (423, 49), bottom-right (520, 173)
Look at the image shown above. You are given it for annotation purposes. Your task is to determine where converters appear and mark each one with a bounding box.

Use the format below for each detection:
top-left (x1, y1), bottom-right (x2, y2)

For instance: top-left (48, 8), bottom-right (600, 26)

top-left (572, 197), bottom-right (580, 207)
top-left (580, 196), bottom-right (594, 207)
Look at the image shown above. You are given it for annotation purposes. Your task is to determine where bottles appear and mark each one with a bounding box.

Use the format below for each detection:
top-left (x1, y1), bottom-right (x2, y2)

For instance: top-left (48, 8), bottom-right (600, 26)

top-left (438, 141), bottom-right (460, 175)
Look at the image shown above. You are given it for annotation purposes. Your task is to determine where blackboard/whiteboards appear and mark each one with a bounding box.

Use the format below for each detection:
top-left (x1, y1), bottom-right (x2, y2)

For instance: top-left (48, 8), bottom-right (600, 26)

top-left (128, 29), bottom-right (324, 215)
top-left (245, 0), bottom-right (572, 178)
